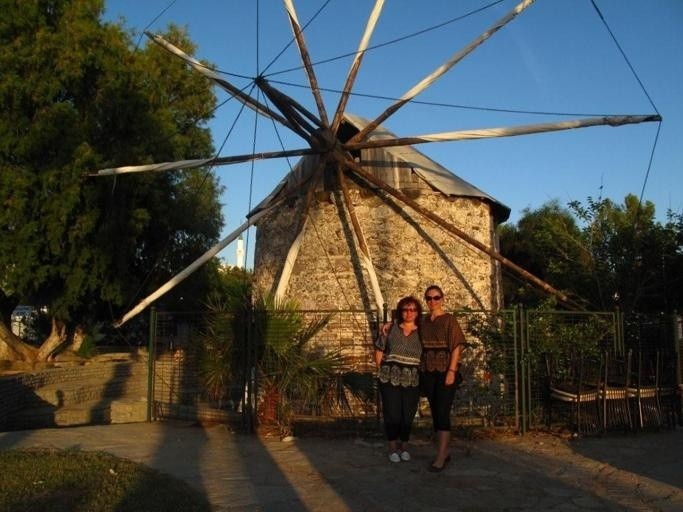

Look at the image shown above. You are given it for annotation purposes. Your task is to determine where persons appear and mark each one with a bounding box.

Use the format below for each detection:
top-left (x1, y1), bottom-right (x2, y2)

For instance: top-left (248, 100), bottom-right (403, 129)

top-left (381, 285), bottom-right (467, 471)
top-left (374, 296), bottom-right (422, 463)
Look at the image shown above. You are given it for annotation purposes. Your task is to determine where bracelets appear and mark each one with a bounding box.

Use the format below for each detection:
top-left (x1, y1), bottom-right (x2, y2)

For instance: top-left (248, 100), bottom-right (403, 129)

top-left (448, 368), bottom-right (456, 373)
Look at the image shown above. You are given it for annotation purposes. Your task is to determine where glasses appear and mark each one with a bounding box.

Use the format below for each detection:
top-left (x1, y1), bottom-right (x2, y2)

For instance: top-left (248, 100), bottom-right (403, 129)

top-left (425, 295), bottom-right (442, 301)
top-left (402, 308), bottom-right (417, 313)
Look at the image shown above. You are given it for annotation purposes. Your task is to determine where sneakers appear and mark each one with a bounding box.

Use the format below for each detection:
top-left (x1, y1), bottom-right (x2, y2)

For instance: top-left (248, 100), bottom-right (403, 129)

top-left (389, 453), bottom-right (401, 463)
top-left (401, 451), bottom-right (411, 462)
top-left (429, 454), bottom-right (452, 473)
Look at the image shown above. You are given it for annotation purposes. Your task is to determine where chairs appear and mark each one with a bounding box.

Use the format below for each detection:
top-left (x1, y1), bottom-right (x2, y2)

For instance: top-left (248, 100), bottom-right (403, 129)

top-left (544, 350), bottom-right (675, 435)
